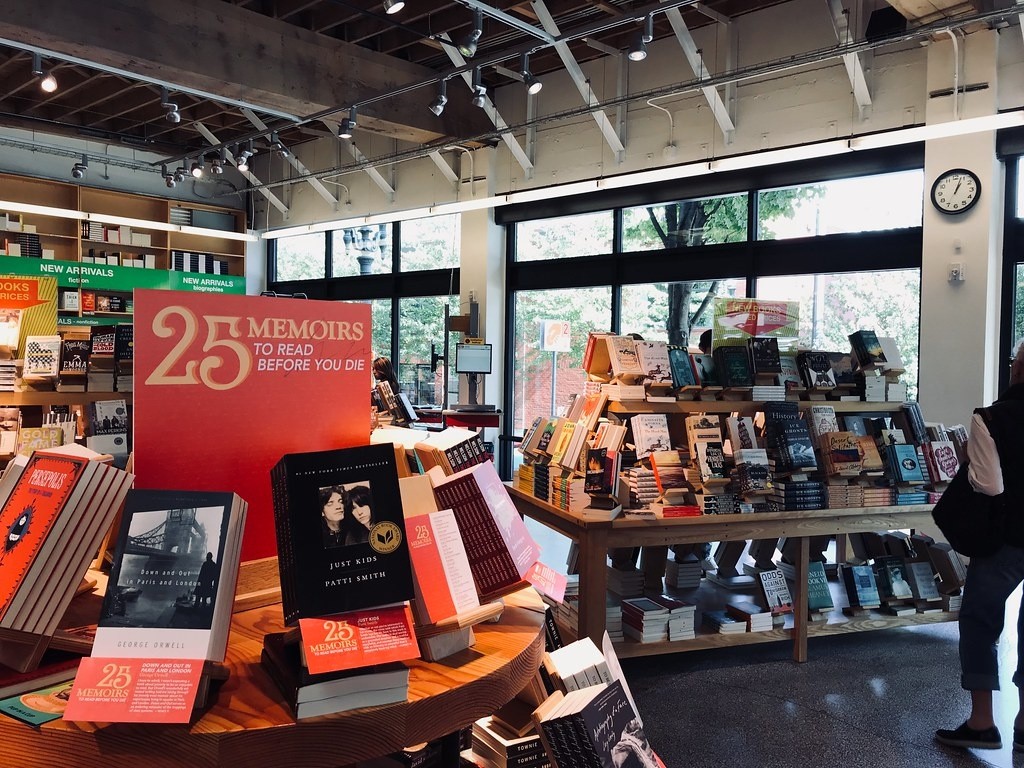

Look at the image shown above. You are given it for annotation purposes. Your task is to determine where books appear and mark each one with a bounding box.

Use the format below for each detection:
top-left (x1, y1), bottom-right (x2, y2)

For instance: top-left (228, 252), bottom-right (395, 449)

top-left (0, 208), bottom-right (250, 663)
top-left (371, 381), bottom-right (417, 423)
top-left (542, 526), bottom-right (965, 643)
top-left (515, 330), bottom-right (969, 517)
top-left (387, 630), bottom-right (660, 768)
top-left (259, 423), bottom-right (543, 722)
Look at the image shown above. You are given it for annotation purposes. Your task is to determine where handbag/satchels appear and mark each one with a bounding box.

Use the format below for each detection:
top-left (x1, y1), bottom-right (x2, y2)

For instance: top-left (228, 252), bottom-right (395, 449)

top-left (932, 461), bottom-right (1010, 560)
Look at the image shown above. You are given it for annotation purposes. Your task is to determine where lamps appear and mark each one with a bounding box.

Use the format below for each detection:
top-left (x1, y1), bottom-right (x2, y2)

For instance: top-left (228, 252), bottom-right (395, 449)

top-left (30, 0), bottom-right (660, 185)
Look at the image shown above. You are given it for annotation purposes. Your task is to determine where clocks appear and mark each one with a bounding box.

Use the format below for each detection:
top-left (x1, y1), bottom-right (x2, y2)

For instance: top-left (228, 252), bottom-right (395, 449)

top-left (930, 168), bottom-right (982, 215)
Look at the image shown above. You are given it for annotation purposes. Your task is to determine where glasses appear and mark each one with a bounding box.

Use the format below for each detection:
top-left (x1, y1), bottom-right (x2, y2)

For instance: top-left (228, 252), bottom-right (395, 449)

top-left (1006, 356), bottom-right (1024, 368)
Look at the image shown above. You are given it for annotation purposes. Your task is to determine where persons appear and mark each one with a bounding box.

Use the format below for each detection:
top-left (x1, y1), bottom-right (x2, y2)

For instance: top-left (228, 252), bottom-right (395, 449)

top-left (695, 358), bottom-right (709, 382)
top-left (854, 421), bottom-right (859, 435)
top-left (111, 416), bottom-right (119, 427)
top-left (753, 412), bottom-right (767, 437)
top-left (317, 484), bottom-right (347, 547)
top-left (935, 341), bottom-right (1024, 752)
top-left (627, 333), bottom-right (645, 341)
top-left (737, 416), bottom-right (752, 449)
top-left (371, 357), bottom-right (400, 413)
top-left (103, 416), bottom-right (110, 429)
top-left (588, 450), bottom-right (603, 470)
top-left (343, 486), bottom-right (375, 547)
top-left (193, 552), bottom-right (216, 608)
top-left (699, 329), bottom-right (712, 356)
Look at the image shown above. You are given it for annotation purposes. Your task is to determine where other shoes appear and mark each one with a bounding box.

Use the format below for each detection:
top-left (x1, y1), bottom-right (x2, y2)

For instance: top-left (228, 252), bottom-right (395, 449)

top-left (1012, 728), bottom-right (1024, 753)
top-left (935, 720), bottom-right (1002, 749)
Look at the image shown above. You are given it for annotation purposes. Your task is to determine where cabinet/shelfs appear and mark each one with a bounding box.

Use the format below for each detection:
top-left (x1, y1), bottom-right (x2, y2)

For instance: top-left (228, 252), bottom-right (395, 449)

top-left (0, 174), bottom-right (256, 459)
top-left (499, 401), bottom-right (971, 663)
